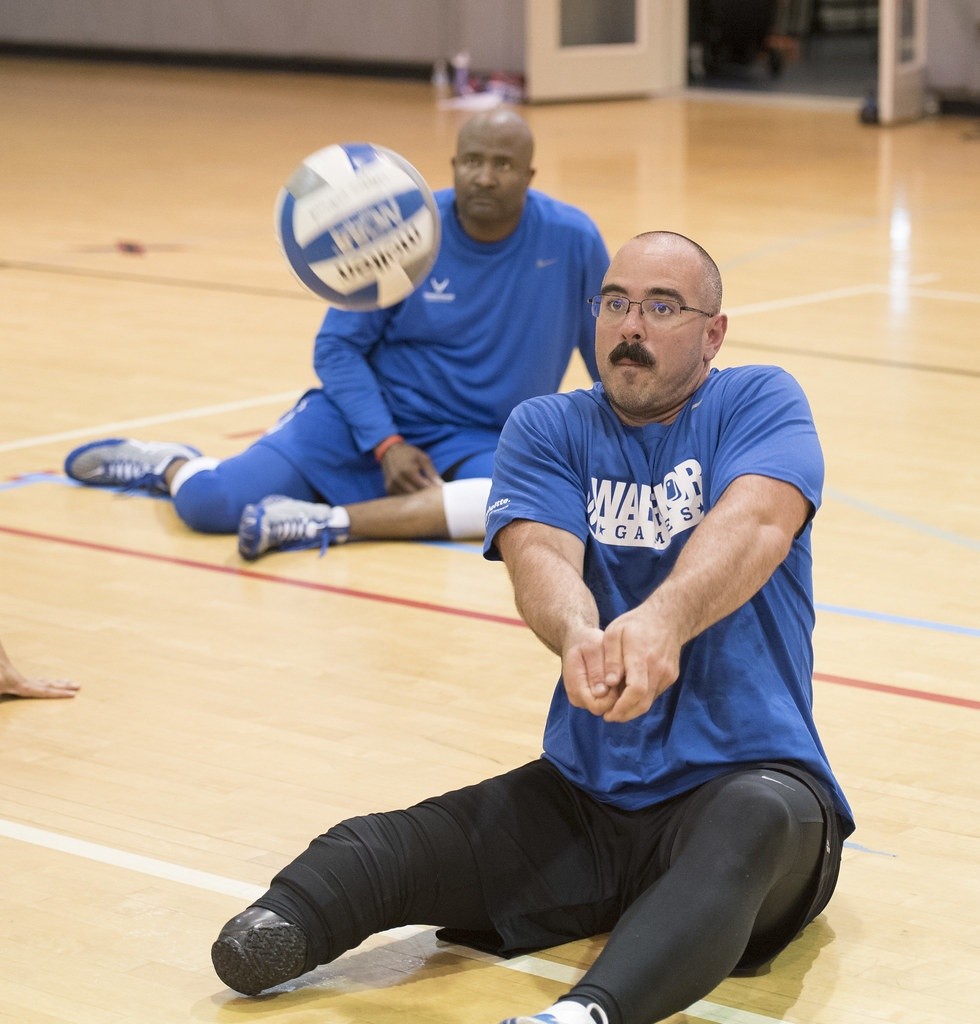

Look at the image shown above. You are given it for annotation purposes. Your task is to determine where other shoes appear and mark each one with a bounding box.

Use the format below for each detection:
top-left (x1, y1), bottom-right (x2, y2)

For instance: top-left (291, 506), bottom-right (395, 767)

top-left (500, 1001), bottom-right (610, 1024)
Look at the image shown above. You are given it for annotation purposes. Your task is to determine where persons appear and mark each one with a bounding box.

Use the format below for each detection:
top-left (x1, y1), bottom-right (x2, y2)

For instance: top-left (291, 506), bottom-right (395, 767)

top-left (64, 107), bottom-right (609, 561)
top-left (211, 231), bottom-right (857, 1024)
top-left (0, 640), bottom-right (80, 698)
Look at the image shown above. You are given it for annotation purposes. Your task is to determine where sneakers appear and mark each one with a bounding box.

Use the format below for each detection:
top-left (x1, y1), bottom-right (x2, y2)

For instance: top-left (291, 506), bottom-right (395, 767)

top-left (66, 440), bottom-right (201, 493)
top-left (239, 495), bottom-right (351, 562)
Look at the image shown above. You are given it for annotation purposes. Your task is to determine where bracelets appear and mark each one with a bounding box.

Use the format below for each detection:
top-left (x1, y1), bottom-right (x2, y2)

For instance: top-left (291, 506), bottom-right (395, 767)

top-left (374, 435), bottom-right (404, 462)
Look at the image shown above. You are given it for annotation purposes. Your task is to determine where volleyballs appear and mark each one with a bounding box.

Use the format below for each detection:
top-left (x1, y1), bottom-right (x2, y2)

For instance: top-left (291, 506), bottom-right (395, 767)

top-left (280, 142), bottom-right (443, 313)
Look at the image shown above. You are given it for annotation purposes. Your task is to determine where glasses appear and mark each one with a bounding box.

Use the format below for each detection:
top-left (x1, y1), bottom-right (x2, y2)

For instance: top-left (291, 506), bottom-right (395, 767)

top-left (588, 295), bottom-right (710, 325)
